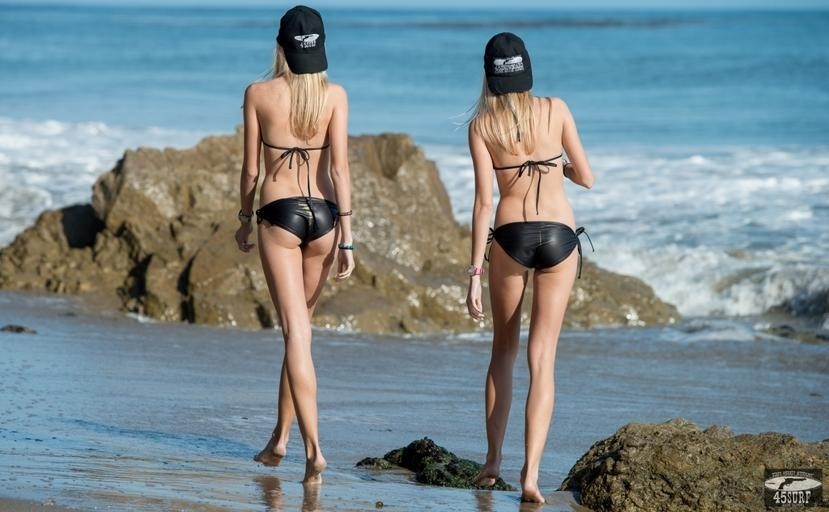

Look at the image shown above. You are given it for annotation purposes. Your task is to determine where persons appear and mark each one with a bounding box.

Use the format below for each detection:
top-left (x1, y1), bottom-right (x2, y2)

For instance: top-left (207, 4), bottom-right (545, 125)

top-left (236, 5), bottom-right (356, 484)
top-left (466, 29), bottom-right (594, 505)
top-left (253, 472), bottom-right (323, 512)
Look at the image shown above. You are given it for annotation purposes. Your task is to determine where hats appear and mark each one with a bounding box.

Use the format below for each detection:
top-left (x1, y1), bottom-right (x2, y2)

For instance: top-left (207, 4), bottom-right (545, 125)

top-left (483, 33), bottom-right (534, 95)
top-left (279, 5), bottom-right (328, 75)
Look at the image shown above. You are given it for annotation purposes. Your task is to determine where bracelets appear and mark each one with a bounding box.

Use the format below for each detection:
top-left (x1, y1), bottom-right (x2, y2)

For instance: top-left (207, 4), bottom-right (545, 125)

top-left (339, 242), bottom-right (355, 251)
top-left (340, 211), bottom-right (351, 216)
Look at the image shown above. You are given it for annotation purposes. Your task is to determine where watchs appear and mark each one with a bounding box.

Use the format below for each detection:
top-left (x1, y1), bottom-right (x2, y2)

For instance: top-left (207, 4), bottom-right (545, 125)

top-left (468, 265), bottom-right (483, 277)
top-left (238, 207), bottom-right (255, 224)
